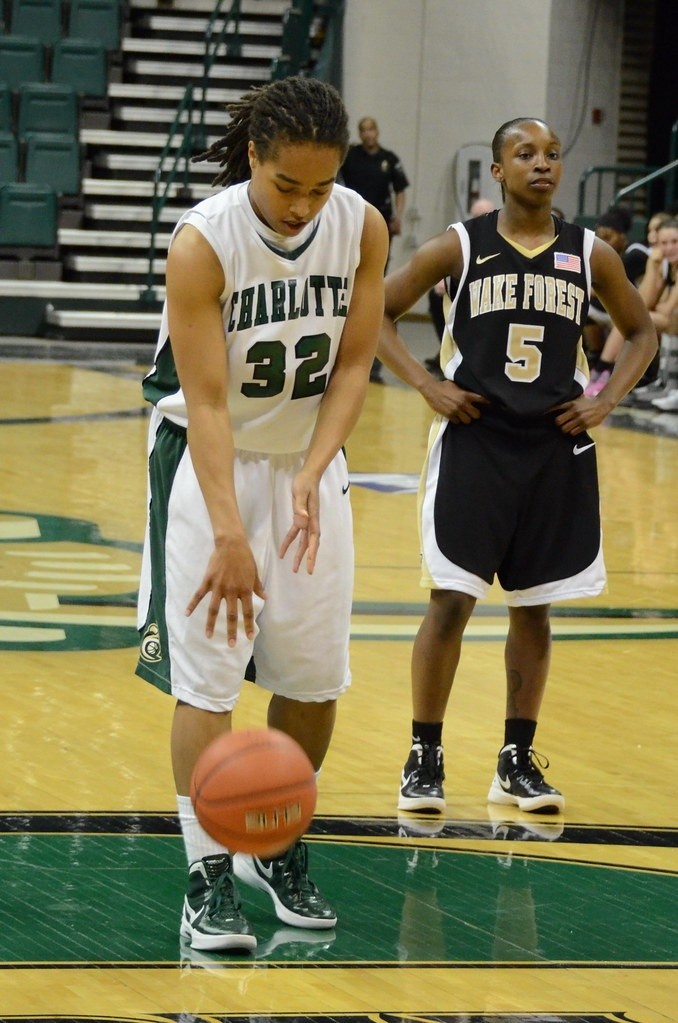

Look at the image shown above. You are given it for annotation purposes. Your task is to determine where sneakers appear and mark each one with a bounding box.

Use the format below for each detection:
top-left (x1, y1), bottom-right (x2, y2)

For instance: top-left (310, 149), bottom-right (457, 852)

top-left (232, 840), bottom-right (337, 928)
top-left (399, 741), bottom-right (446, 811)
top-left (178, 852), bottom-right (257, 950)
top-left (489, 743), bottom-right (564, 812)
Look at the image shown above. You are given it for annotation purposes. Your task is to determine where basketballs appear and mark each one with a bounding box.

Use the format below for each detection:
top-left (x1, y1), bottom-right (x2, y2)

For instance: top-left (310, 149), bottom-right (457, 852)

top-left (190, 726), bottom-right (318, 860)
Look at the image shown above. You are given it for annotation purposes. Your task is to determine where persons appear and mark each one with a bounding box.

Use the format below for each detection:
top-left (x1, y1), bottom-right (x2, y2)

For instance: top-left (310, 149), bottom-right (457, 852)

top-left (375, 116), bottom-right (658, 815)
top-left (421, 197), bottom-right (678, 413)
top-left (334, 117), bottom-right (410, 384)
top-left (134, 75), bottom-right (391, 950)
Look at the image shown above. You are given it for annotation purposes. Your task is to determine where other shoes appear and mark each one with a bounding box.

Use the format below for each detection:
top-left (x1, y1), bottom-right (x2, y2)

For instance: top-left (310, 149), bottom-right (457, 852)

top-left (583, 367), bottom-right (598, 389)
top-left (638, 379), bottom-right (676, 401)
top-left (651, 388), bottom-right (678, 411)
top-left (424, 348), bottom-right (442, 373)
top-left (583, 370), bottom-right (610, 397)
top-left (632, 378), bottom-right (664, 394)
